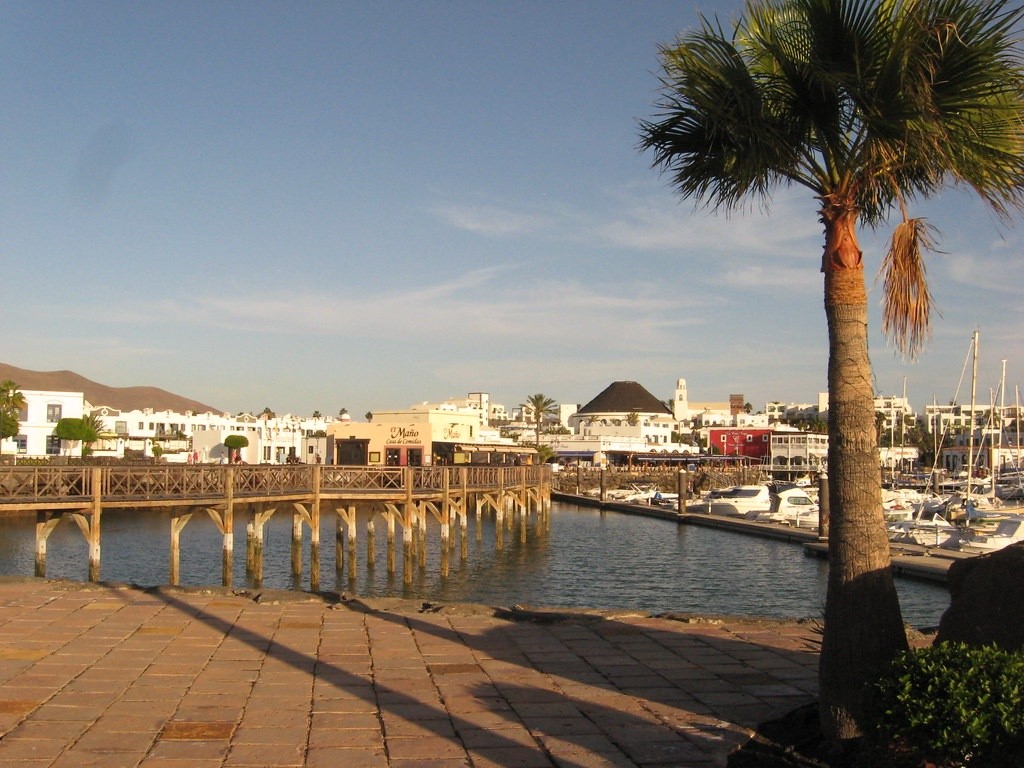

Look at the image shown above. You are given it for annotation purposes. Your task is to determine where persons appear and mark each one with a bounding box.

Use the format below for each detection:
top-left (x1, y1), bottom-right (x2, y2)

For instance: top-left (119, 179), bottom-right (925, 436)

top-left (513, 453), bottom-right (577, 473)
top-left (187, 449), bottom-right (203, 465)
top-left (660, 461), bottom-right (767, 481)
top-left (286, 455), bottom-right (300, 464)
top-left (219, 452), bottom-right (226, 465)
top-left (316, 455), bottom-right (322, 464)
top-left (693, 463), bottom-right (705, 494)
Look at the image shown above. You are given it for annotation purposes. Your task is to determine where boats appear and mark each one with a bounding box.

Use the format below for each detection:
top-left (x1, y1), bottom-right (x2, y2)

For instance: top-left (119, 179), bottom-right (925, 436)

top-left (582, 481), bottom-right (820, 528)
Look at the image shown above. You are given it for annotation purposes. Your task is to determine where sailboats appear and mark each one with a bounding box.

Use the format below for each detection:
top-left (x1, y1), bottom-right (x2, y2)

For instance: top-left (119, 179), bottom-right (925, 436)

top-left (882, 330), bottom-right (1024, 554)
